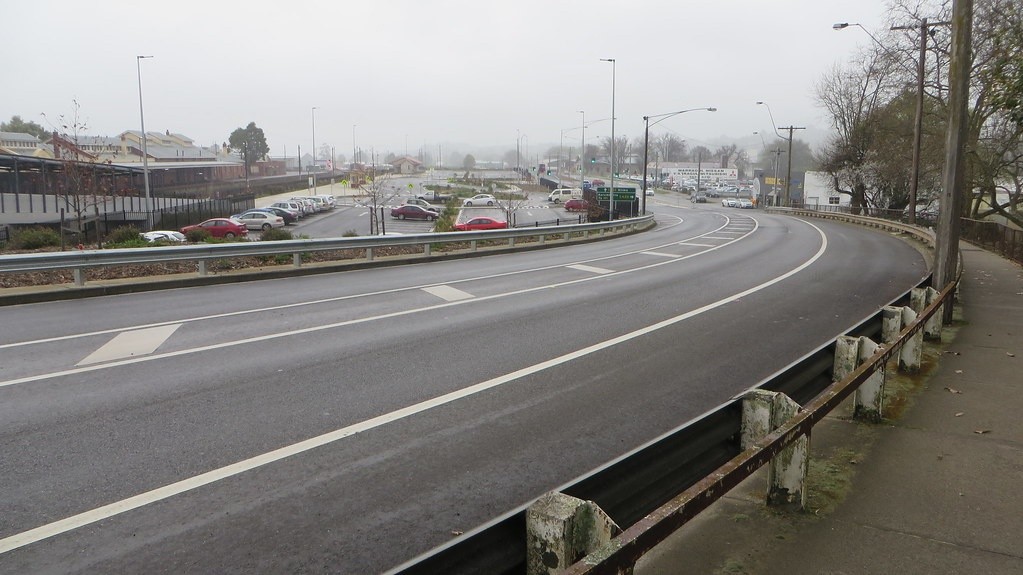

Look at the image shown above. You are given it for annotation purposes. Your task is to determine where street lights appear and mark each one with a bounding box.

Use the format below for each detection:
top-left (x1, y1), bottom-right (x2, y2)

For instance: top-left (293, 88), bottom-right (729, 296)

top-left (311, 107), bottom-right (317, 196)
top-left (756, 101), bottom-right (792, 206)
top-left (353, 124), bottom-right (360, 170)
top-left (579, 111), bottom-right (584, 224)
top-left (640, 108), bottom-right (717, 215)
top-left (559, 126), bottom-right (588, 188)
top-left (136, 55), bottom-right (155, 231)
top-left (832, 23), bottom-right (924, 224)
top-left (753, 132), bottom-right (778, 206)
top-left (600, 58), bottom-right (615, 221)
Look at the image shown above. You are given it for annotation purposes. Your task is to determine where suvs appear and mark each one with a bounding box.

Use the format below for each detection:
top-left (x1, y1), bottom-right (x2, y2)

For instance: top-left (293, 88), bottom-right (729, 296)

top-left (401, 197), bottom-right (442, 213)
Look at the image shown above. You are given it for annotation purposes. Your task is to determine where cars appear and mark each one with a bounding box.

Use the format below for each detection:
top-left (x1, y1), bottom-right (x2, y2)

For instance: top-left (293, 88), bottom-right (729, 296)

top-left (733, 199), bottom-right (753, 209)
top-left (451, 216), bottom-right (507, 232)
top-left (390, 205), bottom-right (440, 221)
top-left (691, 192), bottom-right (707, 203)
top-left (584, 180), bottom-right (605, 189)
top-left (141, 194), bottom-right (337, 243)
top-left (564, 199), bottom-right (588, 212)
top-left (463, 194), bottom-right (496, 207)
top-left (617, 171), bottom-right (753, 196)
top-left (721, 198), bottom-right (737, 207)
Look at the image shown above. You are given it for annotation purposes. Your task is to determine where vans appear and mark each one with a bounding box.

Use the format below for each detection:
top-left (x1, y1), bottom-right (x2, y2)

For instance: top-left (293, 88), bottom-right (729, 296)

top-left (547, 189), bottom-right (583, 204)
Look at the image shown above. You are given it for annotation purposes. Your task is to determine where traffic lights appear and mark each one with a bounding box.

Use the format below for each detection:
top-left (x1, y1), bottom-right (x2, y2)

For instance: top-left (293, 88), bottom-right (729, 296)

top-left (533, 158), bottom-right (617, 177)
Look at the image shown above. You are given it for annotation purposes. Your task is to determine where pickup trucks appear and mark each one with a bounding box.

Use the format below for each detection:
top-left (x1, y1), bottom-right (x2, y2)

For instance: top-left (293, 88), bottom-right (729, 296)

top-left (415, 189), bottom-right (453, 204)
